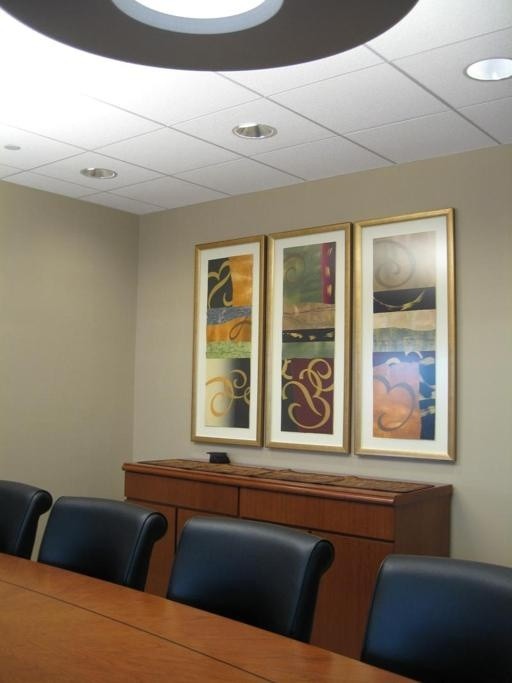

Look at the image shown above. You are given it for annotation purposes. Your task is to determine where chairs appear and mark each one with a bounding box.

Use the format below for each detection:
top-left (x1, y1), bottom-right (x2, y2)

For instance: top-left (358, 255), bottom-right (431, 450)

top-left (0, 478), bottom-right (53, 560)
top-left (37, 496), bottom-right (169, 591)
top-left (165, 514), bottom-right (334, 643)
top-left (361, 554), bottom-right (511, 683)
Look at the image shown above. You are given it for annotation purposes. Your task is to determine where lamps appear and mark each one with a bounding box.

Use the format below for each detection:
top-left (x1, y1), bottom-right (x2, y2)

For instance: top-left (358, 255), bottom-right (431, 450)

top-left (1, 0), bottom-right (419, 73)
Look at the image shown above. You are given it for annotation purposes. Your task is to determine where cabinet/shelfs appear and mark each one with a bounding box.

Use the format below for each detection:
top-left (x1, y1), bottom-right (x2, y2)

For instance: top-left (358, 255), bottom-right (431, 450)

top-left (122, 458), bottom-right (453, 661)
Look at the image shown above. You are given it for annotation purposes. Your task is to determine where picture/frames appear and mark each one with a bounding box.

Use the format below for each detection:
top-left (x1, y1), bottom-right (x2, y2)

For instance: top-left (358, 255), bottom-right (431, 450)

top-left (262, 222), bottom-right (353, 454)
top-left (350, 207), bottom-right (455, 462)
top-left (190, 233), bottom-right (267, 447)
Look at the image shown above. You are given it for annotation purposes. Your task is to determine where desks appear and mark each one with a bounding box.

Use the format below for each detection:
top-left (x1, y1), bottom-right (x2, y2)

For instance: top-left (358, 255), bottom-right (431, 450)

top-left (0, 551), bottom-right (419, 683)
